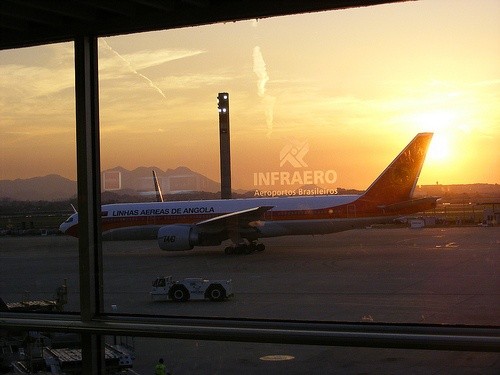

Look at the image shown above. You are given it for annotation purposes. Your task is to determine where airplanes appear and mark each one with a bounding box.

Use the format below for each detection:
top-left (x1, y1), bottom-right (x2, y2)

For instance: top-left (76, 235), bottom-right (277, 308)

top-left (58, 133), bottom-right (443, 254)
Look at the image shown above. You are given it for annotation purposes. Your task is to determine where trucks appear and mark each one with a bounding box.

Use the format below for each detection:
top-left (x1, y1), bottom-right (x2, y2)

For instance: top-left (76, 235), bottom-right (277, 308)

top-left (149, 274), bottom-right (233, 305)
